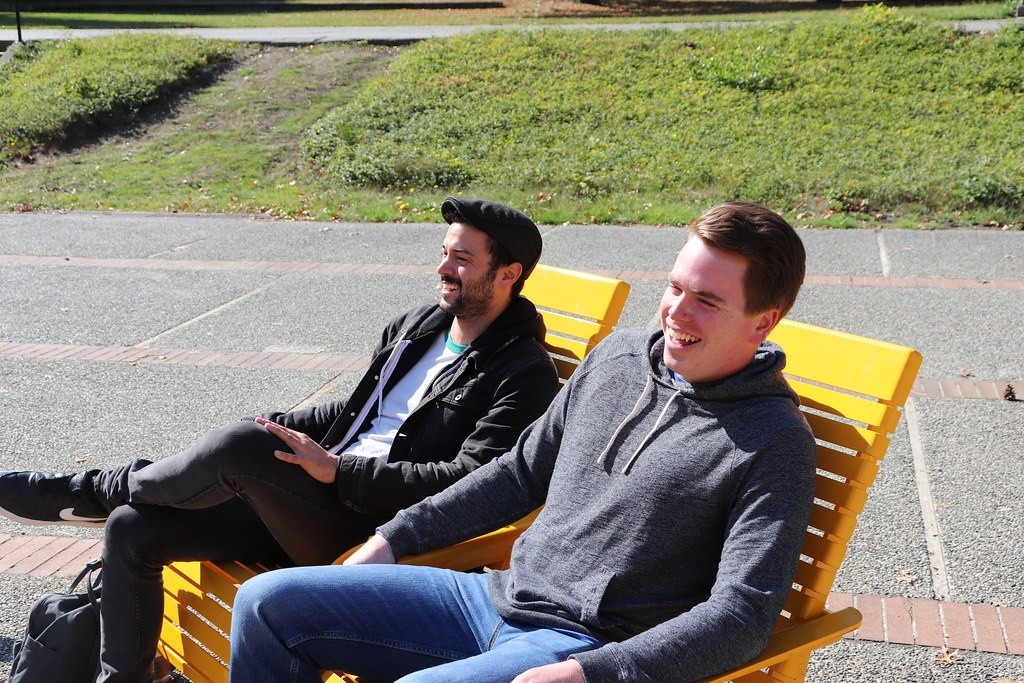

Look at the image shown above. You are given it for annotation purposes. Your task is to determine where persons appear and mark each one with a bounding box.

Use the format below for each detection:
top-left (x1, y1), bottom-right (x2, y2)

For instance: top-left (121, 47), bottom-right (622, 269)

top-left (234, 204), bottom-right (820, 683)
top-left (0, 195), bottom-right (560, 683)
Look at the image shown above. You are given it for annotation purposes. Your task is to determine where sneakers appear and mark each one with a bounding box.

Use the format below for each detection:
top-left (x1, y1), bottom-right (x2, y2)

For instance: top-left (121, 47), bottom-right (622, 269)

top-left (0, 469), bottom-right (109, 528)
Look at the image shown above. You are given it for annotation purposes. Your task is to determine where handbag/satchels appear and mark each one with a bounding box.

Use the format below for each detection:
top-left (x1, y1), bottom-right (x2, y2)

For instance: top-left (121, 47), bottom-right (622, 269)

top-left (9, 557), bottom-right (104, 683)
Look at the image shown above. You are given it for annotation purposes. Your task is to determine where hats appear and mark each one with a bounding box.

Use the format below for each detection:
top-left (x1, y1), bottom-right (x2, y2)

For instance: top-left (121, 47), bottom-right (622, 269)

top-left (442, 196), bottom-right (542, 280)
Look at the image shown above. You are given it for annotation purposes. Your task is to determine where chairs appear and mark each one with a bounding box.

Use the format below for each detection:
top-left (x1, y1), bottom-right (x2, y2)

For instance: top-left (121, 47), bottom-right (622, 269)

top-left (155, 262), bottom-right (632, 683)
top-left (317, 308), bottom-right (924, 683)
